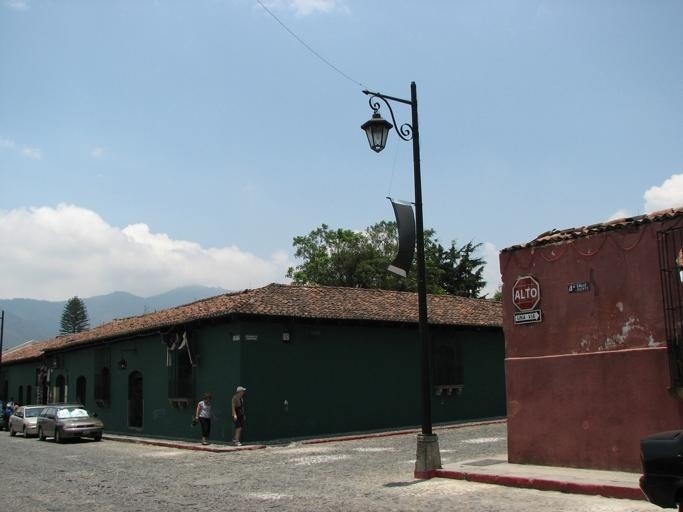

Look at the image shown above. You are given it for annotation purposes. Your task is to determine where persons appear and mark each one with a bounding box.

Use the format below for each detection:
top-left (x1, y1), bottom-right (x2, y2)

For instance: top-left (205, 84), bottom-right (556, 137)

top-left (196, 392), bottom-right (217, 445)
top-left (0, 396), bottom-right (23, 417)
top-left (230, 386), bottom-right (247, 446)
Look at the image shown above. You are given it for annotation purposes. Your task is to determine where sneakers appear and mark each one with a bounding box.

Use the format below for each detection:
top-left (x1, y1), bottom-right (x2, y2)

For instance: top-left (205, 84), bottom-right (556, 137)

top-left (233, 438), bottom-right (242, 446)
top-left (201, 439), bottom-right (211, 445)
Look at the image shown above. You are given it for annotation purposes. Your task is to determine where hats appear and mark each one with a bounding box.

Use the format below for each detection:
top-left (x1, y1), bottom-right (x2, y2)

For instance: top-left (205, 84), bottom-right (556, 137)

top-left (236, 386), bottom-right (246, 392)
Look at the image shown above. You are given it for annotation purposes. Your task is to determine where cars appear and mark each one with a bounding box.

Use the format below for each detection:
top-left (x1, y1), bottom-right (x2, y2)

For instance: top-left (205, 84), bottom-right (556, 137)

top-left (0, 401), bottom-right (103, 443)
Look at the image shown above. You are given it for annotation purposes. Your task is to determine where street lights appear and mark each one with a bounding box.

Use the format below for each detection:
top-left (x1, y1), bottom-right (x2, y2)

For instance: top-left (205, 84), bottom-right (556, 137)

top-left (360, 80), bottom-right (441, 479)
